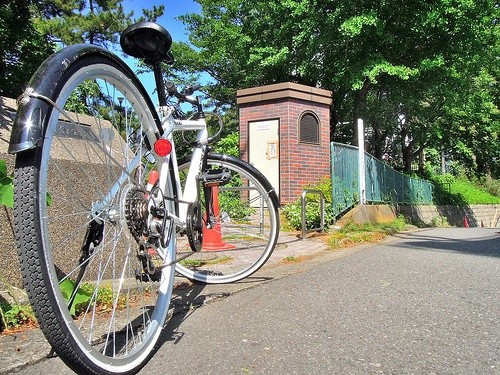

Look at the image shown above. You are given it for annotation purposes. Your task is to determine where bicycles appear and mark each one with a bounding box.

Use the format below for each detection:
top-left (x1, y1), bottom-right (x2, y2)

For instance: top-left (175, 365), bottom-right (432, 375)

top-left (7, 20), bottom-right (281, 375)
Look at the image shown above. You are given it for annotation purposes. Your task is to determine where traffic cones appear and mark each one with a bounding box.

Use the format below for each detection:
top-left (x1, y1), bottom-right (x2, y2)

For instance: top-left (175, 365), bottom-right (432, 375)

top-left (143, 170), bottom-right (177, 255)
top-left (186, 183), bottom-right (237, 250)
